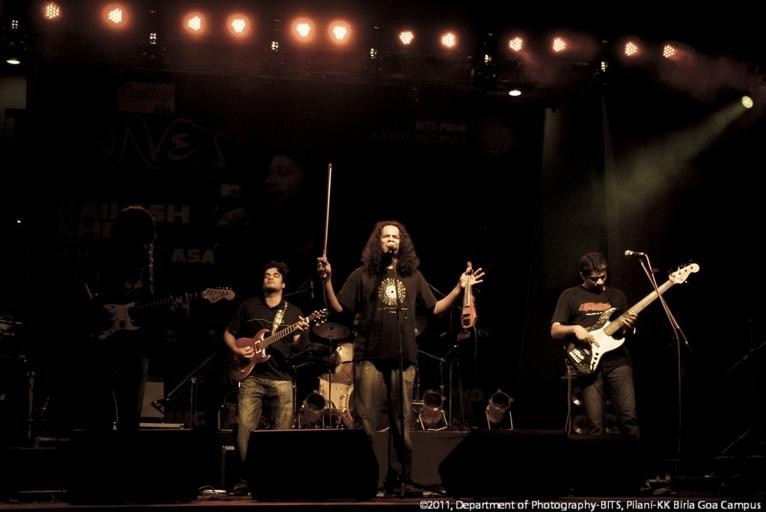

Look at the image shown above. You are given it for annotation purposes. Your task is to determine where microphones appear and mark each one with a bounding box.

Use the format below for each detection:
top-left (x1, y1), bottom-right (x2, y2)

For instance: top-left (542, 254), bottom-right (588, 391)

top-left (387, 242), bottom-right (397, 256)
top-left (624, 250), bottom-right (646, 258)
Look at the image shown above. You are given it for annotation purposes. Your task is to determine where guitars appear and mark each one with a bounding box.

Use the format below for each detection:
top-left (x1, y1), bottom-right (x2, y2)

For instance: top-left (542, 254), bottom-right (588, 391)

top-left (228, 309), bottom-right (329, 381)
top-left (95, 286), bottom-right (235, 340)
top-left (565, 262), bottom-right (700, 375)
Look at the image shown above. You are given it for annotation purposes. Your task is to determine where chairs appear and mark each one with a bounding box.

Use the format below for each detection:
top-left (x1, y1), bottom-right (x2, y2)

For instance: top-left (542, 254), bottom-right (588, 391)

top-left (563, 345), bottom-right (630, 438)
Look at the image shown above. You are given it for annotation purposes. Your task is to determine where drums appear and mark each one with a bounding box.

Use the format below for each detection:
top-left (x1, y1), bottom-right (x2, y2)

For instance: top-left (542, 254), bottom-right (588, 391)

top-left (340, 383), bottom-right (390, 432)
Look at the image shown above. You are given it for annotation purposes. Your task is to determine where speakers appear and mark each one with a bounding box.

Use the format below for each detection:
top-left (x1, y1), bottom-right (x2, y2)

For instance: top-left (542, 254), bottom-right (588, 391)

top-left (438, 430), bottom-right (573, 498)
top-left (68, 429), bottom-right (201, 505)
top-left (244, 429), bottom-right (379, 501)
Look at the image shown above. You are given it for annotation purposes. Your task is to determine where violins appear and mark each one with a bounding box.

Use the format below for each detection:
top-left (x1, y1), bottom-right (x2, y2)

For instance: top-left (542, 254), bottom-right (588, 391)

top-left (461, 261), bottom-right (478, 328)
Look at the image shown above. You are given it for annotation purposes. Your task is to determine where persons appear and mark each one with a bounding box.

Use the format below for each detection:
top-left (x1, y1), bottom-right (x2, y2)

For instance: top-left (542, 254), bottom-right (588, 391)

top-left (553, 251), bottom-right (652, 435)
top-left (229, 263), bottom-right (309, 493)
top-left (318, 221), bottom-right (482, 495)
top-left (82, 208), bottom-right (191, 432)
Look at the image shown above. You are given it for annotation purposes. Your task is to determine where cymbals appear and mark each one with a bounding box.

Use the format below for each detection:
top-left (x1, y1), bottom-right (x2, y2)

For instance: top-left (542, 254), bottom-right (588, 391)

top-left (414, 316), bottom-right (426, 336)
top-left (313, 321), bottom-right (351, 339)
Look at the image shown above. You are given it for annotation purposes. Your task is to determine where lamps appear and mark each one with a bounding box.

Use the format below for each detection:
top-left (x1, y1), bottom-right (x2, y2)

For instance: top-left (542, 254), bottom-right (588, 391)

top-left (150, 352), bottom-right (217, 427)
